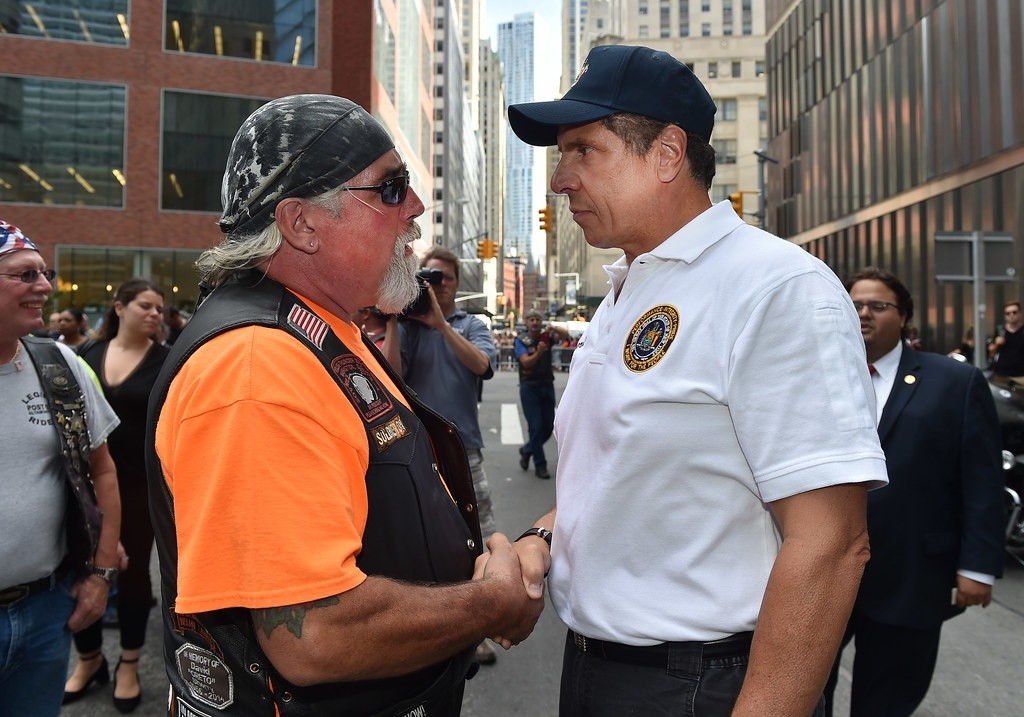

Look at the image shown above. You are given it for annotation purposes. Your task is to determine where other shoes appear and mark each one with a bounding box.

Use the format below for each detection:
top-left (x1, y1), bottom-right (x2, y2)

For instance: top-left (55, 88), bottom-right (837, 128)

top-left (519, 447), bottom-right (530, 470)
top-left (475, 639), bottom-right (497, 665)
top-left (536, 470), bottom-right (550, 479)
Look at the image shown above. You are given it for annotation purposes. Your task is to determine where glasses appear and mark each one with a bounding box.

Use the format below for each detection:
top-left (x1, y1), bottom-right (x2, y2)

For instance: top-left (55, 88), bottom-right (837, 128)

top-left (336, 171), bottom-right (410, 207)
top-left (1005, 311), bottom-right (1019, 315)
top-left (0, 268), bottom-right (57, 283)
top-left (854, 301), bottom-right (899, 312)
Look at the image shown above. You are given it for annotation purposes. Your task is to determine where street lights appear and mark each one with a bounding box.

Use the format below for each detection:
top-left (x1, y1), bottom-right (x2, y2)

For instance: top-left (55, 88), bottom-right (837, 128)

top-left (553, 273), bottom-right (580, 310)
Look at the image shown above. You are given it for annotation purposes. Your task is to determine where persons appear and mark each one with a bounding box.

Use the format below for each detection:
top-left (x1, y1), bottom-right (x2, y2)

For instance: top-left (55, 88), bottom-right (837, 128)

top-left (147, 97), bottom-right (547, 717)
top-left (351, 305), bottom-right (387, 350)
top-left (157, 304), bottom-right (193, 348)
top-left (378, 247), bottom-right (498, 665)
top-left (469, 44), bottom-right (888, 717)
top-left (542, 325), bottom-right (584, 372)
top-left (0, 218), bottom-right (122, 717)
top-left (62, 279), bottom-right (175, 714)
top-left (811, 268), bottom-right (1003, 717)
top-left (31, 305), bottom-right (94, 355)
top-left (949, 301), bottom-right (1024, 378)
top-left (513, 309), bottom-right (570, 478)
top-left (904, 325), bottom-right (922, 349)
top-left (492, 328), bottom-right (520, 372)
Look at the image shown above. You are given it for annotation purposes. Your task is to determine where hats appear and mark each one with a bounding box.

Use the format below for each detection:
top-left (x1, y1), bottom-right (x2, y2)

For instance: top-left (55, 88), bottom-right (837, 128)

top-left (507, 46), bottom-right (717, 147)
top-left (217, 93), bottom-right (399, 235)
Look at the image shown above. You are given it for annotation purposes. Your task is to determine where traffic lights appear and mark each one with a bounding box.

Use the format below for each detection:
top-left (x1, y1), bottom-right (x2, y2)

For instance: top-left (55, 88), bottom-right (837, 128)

top-left (477, 240), bottom-right (488, 258)
top-left (539, 204), bottom-right (552, 231)
top-left (487, 240), bottom-right (498, 258)
top-left (729, 192), bottom-right (743, 220)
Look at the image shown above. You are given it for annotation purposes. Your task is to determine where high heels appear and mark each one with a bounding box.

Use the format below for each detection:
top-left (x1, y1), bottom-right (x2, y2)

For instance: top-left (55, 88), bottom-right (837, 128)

top-left (62, 653), bottom-right (110, 703)
top-left (113, 655), bottom-right (142, 715)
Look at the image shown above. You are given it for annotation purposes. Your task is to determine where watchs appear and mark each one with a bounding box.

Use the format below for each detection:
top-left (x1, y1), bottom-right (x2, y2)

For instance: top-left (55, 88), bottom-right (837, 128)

top-left (93, 566), bottom-right (119, 583)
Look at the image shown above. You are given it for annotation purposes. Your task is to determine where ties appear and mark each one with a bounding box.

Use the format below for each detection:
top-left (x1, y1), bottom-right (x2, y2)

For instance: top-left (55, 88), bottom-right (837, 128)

top-left (867, 363), bottom-right (877, 375)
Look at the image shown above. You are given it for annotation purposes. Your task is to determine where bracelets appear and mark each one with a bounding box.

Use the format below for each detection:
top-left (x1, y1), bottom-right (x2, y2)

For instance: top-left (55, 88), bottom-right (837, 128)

top-left (514, 528), bottom-right (553, 545)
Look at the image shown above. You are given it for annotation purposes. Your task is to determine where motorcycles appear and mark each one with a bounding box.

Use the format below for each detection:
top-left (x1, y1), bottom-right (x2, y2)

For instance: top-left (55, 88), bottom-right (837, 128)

top-left (987, 382), bottom-right (1024, 572)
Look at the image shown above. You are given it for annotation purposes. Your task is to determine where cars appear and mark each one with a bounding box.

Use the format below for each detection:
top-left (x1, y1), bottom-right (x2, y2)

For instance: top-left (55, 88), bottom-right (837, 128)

top-left (541, 321), bottom-right (590, 372)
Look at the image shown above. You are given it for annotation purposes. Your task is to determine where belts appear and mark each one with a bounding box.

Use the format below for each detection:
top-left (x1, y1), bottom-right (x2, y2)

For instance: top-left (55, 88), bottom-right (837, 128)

top-left (571, 632), bottom-right (752, 662)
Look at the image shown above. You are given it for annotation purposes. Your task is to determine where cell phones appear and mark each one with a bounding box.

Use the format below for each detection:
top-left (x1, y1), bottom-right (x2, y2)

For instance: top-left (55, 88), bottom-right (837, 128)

top-left (541, 332), bottom-right (550, 348)
